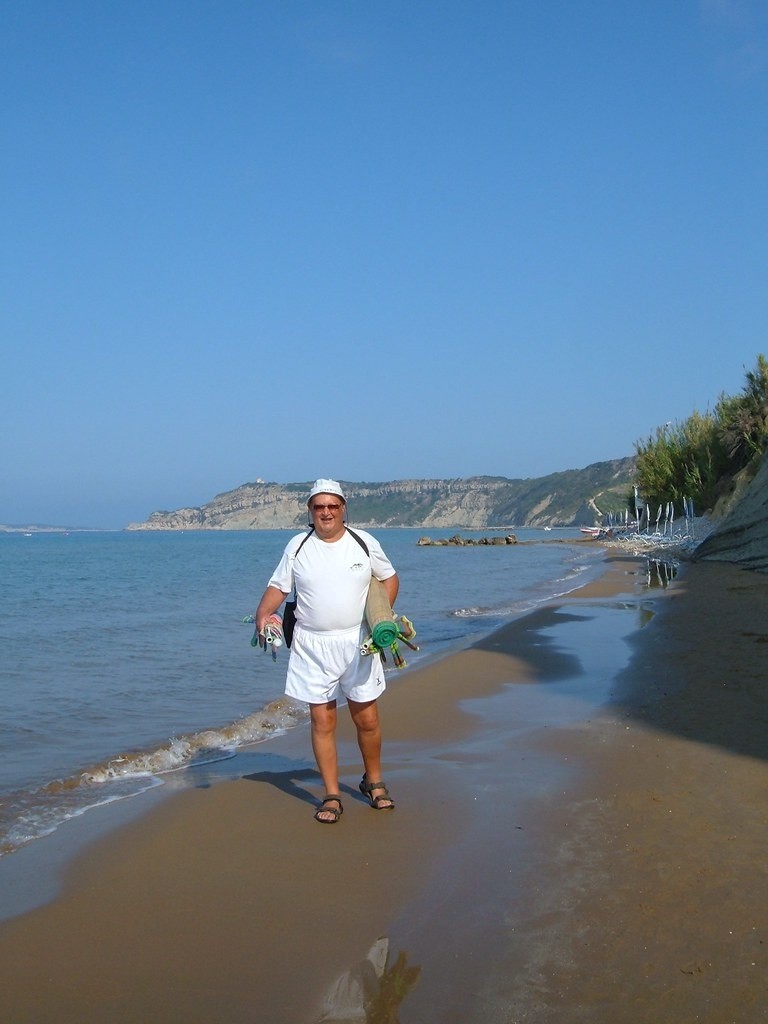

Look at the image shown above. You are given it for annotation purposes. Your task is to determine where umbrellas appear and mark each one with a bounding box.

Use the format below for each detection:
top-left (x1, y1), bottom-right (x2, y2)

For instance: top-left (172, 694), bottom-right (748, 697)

top-left (625, 509), bottom-right (628, 532)
top-left (656, 504), bottom-right (662, 532)
top-left (683, 497), bottom-right (689, 534)
top-left (647, 560), bottom-right (673, 586)
top-left (606, 510), bottom-right (612, 527)
top-left (689, 497), bottom-right (694, 538)
top-left (636, 507), bottom-right (639, 533)
top-left (647, 504), bottom-right (650, 532)
top-left (664, 502), bottom-right (674, 536)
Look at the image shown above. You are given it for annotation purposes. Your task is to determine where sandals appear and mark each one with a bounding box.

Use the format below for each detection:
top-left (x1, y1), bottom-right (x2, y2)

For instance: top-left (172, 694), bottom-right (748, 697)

top-left (314, 794), bottom-right (343, 823)
top-left (359, 773), bottom-right (395, 810)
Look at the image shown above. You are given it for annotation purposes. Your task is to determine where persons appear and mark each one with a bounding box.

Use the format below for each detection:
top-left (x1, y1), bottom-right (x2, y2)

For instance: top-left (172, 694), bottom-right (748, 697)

top-left (255, 478), bottom-right (399, 824)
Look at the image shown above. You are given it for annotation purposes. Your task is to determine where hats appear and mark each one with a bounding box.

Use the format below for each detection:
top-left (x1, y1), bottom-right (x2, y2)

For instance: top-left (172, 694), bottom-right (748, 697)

top-left (307, 478), bottom-right (347, 507)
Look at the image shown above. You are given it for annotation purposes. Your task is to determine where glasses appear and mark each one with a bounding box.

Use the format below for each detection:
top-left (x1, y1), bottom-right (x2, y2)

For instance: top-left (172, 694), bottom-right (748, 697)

top-left (309, 501), bottom-right (343, 512)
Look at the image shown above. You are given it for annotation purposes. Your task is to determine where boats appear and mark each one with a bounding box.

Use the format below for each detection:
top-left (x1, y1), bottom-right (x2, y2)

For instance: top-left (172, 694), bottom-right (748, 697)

top-left (580, 529), bottom-right (598, 533)
top-left (544, 527), bottom-right (551, 532)
